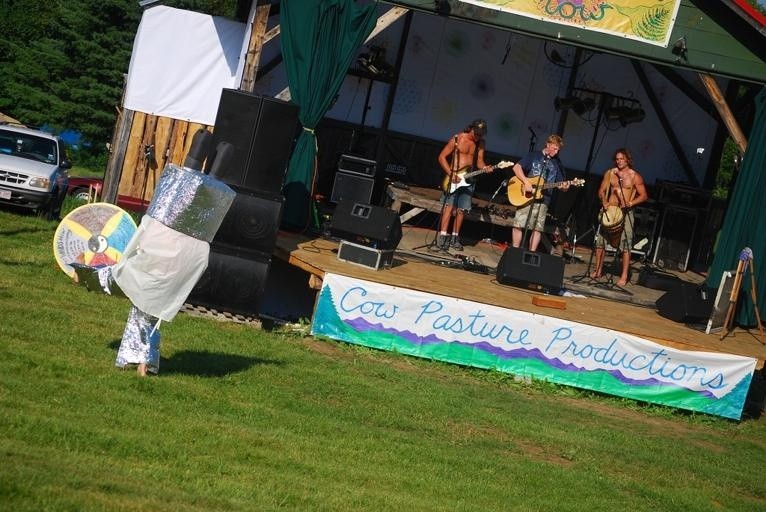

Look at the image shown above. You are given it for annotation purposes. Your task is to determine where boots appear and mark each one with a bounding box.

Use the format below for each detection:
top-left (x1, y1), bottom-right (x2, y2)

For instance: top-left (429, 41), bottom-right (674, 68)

top-left (439, 235), bottom-right (463, 251)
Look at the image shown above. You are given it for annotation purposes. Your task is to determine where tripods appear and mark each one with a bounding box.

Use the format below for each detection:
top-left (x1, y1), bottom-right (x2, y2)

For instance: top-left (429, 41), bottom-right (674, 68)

top-left (413, 146), bottom-right (458, 259)
top-left (572, 181), bottom-right (635, 295)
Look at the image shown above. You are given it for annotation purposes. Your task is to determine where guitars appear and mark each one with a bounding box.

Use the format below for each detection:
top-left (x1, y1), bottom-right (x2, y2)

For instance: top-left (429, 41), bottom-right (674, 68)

top-left (443, 160), bottom-right (516, 196)
top-left (508, 174), bottom-right (587, 208)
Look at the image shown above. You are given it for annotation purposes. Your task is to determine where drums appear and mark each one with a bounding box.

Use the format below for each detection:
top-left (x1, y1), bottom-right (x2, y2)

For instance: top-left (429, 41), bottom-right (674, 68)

top-left (601, 205), bottom-right (624, 235)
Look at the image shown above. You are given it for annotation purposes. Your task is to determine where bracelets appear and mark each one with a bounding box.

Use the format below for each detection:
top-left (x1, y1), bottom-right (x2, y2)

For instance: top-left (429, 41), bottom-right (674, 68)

top-left (628, 202), bottom-right (633, 208)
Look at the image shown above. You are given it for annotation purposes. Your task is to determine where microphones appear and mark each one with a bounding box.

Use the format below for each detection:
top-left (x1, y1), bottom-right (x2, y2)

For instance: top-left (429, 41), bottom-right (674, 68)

top-left (454, 134), bottom-right (459, 146)
top-left (544, 150), bottom-right (550, 160)
top-left (613, 170), bottom-right (622, 182)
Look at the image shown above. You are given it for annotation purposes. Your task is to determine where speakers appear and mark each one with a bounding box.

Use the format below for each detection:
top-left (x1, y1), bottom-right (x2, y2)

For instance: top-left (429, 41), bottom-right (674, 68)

top-left (330, 198), bottom-right (402, 250)
top-left (186, 87), bottom-right (301, 316)
top-left (656, 284), bottom-right (708, 323)
top-left (496, 246), bottom-right (565, 295)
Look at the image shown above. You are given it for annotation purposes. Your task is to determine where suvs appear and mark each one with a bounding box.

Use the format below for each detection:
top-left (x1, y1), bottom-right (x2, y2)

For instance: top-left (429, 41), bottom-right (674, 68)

top-left (0, 121), bottom-right (71, 225)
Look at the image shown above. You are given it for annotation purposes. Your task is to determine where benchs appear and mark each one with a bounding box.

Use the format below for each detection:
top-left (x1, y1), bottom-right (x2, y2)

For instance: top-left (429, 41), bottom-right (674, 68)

top-left (387, 184), bottom-right (515, 228)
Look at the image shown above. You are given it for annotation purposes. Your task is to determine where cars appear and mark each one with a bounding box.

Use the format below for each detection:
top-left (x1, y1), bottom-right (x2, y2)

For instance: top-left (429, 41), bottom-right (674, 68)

top-left (65, 174), bottom-right (102, 205)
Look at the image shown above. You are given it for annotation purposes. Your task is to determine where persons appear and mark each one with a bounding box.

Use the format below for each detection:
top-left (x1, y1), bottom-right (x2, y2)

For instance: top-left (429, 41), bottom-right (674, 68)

top-left (438, 117), bottom-right (493, 251)
top-left (512, 135), bottom-right (570, 252)
top-left (585, 149), bottom-right (648, 286)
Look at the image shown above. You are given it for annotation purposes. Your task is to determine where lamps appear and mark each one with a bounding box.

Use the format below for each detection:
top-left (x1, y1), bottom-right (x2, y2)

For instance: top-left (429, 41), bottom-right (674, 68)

top-left (358, 45), bottom-right (398, 79)
top-left (553, 89), bottom-right (645, 127)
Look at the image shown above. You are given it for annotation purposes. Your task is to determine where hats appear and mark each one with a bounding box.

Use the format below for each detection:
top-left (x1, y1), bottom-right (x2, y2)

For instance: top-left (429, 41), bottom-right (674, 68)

top-left (473, 118), bottom-right (487, 136)
